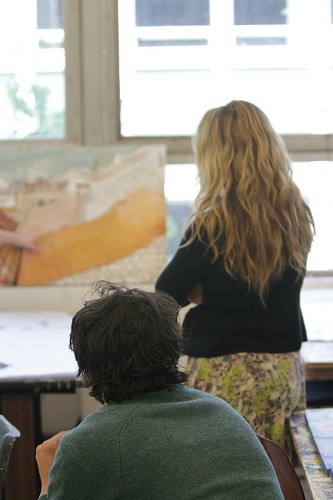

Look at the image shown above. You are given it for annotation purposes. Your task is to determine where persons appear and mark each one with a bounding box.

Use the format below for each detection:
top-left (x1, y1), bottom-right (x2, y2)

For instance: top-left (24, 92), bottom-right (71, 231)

top-left (33, 279), bottom-right (288, 499)
top-left (152, 99), bottom-right (317, 464)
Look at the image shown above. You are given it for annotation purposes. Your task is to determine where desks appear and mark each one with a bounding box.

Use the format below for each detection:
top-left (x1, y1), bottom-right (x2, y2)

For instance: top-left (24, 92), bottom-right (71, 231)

top-left (0, 294), bottom-right (333, 500)
